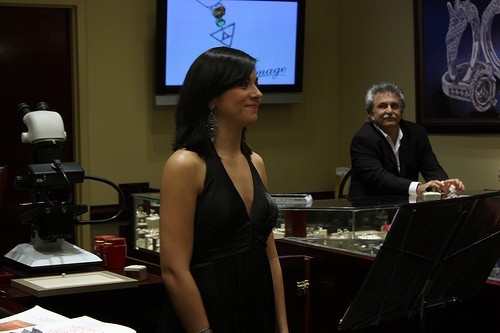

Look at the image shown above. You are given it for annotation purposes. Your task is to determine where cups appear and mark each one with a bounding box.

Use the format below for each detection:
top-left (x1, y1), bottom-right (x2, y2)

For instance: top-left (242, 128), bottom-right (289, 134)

top-left (103, 238), bottom-right (127, 275)
top-left (92, 235), bottom-right (115, 271)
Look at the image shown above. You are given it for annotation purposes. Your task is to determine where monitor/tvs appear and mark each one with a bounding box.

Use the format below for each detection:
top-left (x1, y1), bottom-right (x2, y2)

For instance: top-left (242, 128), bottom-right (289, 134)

top-left (155, 0), bottom-right (305, 93)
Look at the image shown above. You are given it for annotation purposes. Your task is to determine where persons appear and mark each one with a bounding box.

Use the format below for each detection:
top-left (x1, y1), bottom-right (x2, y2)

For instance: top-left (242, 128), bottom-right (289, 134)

top-left (348, 81), bottom-right (465, 199)
top-left (159, 47), bottom-right (288, 333)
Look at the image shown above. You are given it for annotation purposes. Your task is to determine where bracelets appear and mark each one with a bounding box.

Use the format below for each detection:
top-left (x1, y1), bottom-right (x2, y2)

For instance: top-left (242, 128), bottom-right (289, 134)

top-left (200, 328), bottom-right (211, 333)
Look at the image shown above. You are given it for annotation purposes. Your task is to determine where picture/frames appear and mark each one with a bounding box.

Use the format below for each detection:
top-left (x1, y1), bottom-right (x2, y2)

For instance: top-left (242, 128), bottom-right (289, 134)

top-left (413, 0), bottom-right (500, 135)
